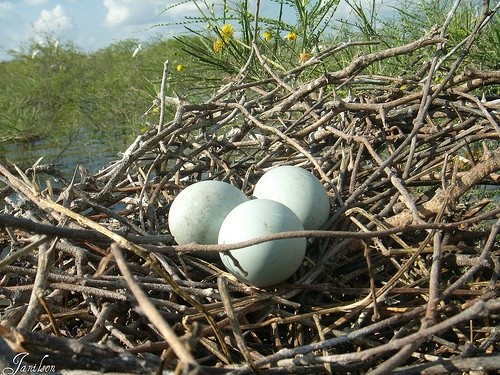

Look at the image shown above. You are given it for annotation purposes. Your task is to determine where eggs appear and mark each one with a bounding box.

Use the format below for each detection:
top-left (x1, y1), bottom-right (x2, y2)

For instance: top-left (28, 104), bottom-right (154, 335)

top-left (251, 165), bottom-right (331, 231)
top-left (216, 199), bottom-right (307, 287)
top-left (167, 180), bottom-right (250, 258)
